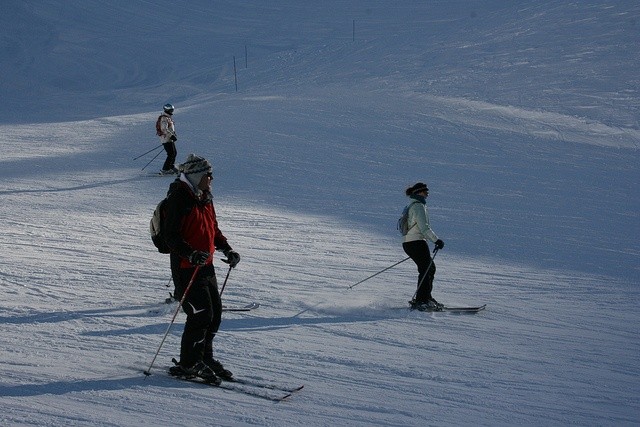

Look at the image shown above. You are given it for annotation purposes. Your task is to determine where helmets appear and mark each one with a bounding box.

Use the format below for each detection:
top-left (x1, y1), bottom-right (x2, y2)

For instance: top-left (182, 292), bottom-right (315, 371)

top-left (163, 103), bottom-right (175, 115)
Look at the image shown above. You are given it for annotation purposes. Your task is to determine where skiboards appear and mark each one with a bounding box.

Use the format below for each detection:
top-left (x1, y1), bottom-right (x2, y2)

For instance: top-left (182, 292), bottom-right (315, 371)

top-left (119, 358), bottom-right (303, 401)
top-left (139, 171), bottom-right (180, 177)
top-left (166, 292), bottom-right (260, 312)
top-left (391, 304), bottom-right (487, 312)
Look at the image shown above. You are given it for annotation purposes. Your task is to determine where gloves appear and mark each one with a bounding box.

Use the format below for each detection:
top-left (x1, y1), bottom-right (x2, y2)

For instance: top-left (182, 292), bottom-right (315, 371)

top-left (221, 250), bottom-right (240, 269)
top-left (171, 135), bottom-right (177, 141)
top-left (188, 250), bottom-right (209, 267)
top-left (433, 239), bottom-right (445, 253)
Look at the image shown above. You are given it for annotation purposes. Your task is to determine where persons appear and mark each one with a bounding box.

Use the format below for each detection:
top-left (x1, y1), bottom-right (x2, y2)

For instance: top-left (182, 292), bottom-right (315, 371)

top-left (402, 183), bottom-right (444, 310)
top-left (167, 153), bottom-right (240, 380)
top-left (159, 104), bottom-right (180, 176)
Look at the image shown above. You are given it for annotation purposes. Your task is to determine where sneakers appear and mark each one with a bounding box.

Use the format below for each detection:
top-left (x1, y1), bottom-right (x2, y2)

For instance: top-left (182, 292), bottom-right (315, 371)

top-left (414, 301), bottom-right (442, 312)
top-left (431, 298), bottom-right (445, 308)
top-left (179, 359), bottom-right (223, 387)
top-left (207, 359), bottom-right (234, 380)
top-left (173, 167), bottom-right (178, 173)
top-left (161, 169), bottom-right (175, 174)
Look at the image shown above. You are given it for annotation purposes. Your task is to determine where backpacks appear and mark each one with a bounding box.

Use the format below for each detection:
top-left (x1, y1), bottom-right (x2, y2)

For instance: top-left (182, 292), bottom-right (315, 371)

top-left (156, 114), bottom-right (167, 136)
top-left (149, 188), bottom-right (183, 254)
top-left (396, 201), bottom-right (422, 236)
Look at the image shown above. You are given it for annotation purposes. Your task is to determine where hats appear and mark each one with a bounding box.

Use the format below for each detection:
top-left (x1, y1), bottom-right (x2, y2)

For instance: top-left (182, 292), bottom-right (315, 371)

top-left (405, 182), bottom-right (429, 196)
top-left (180, 154), bottom-right (214, 192)
